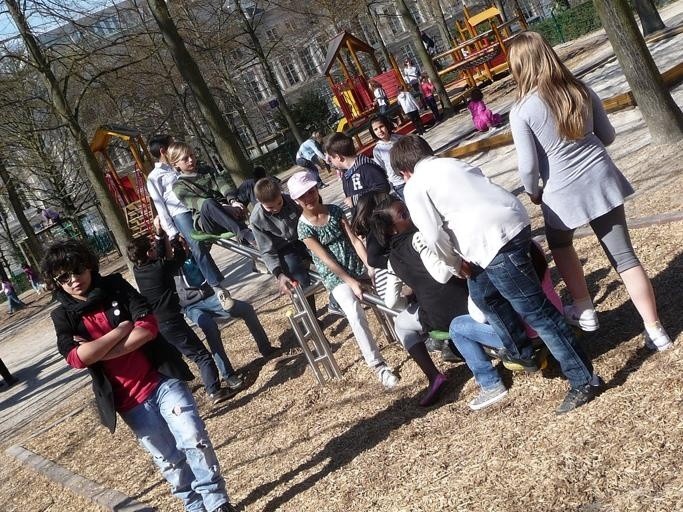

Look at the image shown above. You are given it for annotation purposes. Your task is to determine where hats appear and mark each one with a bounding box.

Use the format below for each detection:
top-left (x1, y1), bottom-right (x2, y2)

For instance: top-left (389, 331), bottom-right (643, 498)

top-left (286, 170), bottom-right (318, 201)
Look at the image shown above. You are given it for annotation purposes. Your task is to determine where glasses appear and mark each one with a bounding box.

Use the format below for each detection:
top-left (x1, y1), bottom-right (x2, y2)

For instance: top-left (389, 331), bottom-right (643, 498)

top-left (53, 264), bottom-right (89, 285)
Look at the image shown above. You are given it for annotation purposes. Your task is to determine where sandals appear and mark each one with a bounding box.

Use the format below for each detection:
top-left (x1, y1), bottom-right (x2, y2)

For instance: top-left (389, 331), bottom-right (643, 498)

top-left (418, 374), bottom-right (448, 406)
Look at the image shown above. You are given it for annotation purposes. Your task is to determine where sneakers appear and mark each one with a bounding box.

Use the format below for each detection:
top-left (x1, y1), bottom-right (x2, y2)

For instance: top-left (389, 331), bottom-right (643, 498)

top-left (564, 304), bottom-right (600, 333)
top-left (378, 366), bottom-right (398, 388)
top-left (643, 331), bottom-right (672, 353)
top-left (217, 289), bottom-right (235, 313)
top-left (502, 354), bottom-right (538, 372)
top-left (264, 346), bottom-right (289, 361)
top-left (251, 259), bottom-right (271, 275)
top-left (320, 183), bottom-right (329, 189)
top-left (441, 344), bottom-right (464, 362)
top-left (424, 338), bottom-right (444, 354)
top-left (209, 373), bottom-right (244, 405)
top-left (489, 127), bottom-right (497, 133)
top-left (468, 383), bottom-right (507, 411)
top-left (554, 374), bottom-right (607, 414)
top-left (327, 294), bottom-right (346, 317)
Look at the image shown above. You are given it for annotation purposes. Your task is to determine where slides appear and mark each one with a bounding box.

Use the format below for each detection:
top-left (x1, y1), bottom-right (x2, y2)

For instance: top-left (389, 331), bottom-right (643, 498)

top-left (333, 93), bottom-right (360, 132)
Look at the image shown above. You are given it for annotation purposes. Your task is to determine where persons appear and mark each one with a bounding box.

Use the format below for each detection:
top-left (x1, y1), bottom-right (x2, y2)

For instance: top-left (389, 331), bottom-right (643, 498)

top-left (39, 239), bottom-right (237, 512)
top-left (0, 33), bottom-right (606, 417)
top-left (507, 31), bottom-right (674, 352)
